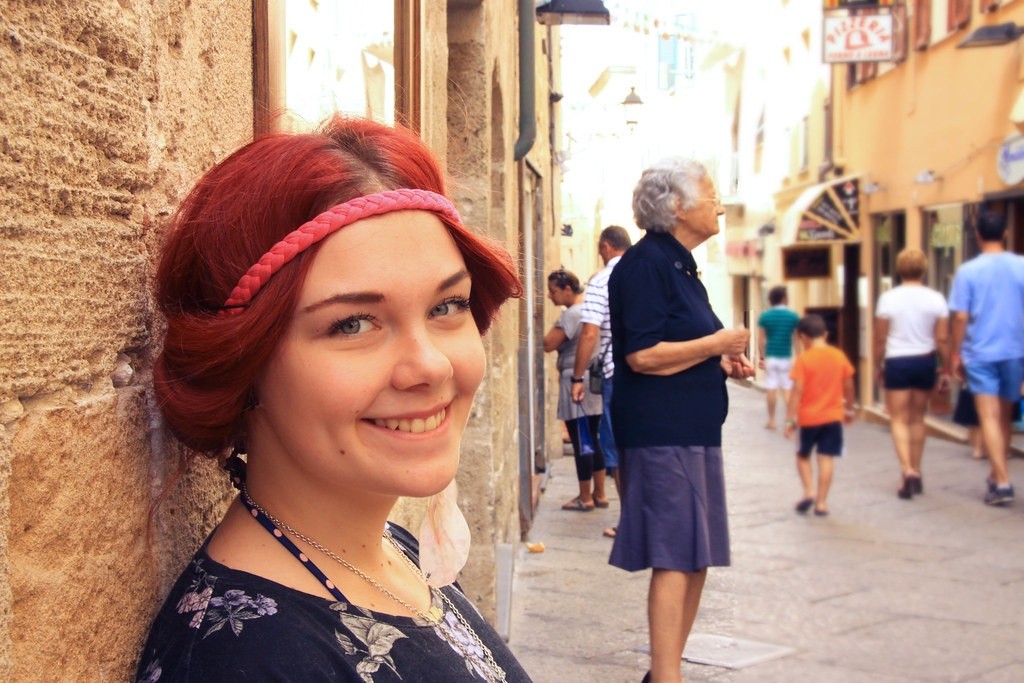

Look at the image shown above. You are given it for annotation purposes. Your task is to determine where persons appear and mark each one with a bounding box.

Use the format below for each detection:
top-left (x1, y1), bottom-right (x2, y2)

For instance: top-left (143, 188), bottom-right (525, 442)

top-left (756, 286), bottom-right (802, 431)
top-left (134, 112), bottom-right (536, 683)
top-left (543, 269), bottom-right (610, 512)
top-left (781, 314), bottom-right (856, 516)
top-left (873, 247), bottom-right (951, 497)
top-left (606, 154), bottom-right (756, 683)
top-left (952, 376), bottom-right (1015, 462)
top-left (948, 211), bottom-right (1023, 507)
top-left (569, 224), bottom-right (634, 537)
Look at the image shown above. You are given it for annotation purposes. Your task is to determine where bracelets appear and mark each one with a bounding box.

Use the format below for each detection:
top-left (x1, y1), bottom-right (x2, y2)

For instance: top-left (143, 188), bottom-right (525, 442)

top-left (785, 417), bottom-right (798, 424)
top-left (759, 357), bottom-right (764, 360)
top-left (570, 377), bottom-right (584, 384)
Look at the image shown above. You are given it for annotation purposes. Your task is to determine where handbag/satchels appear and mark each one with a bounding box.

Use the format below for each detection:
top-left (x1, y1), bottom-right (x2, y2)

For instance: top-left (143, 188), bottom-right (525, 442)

top-left (575, 399), bottom-right (595, 457)
top-left (588, 355), bottom-right (603, 394)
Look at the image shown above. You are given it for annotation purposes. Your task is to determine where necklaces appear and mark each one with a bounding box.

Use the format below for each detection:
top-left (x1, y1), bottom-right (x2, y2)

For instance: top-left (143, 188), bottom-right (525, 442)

top-left (241, 480), bottom-right (512, 683)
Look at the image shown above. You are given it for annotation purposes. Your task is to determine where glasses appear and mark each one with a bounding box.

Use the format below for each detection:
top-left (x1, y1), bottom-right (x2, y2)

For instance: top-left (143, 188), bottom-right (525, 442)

top-left (702, 194), bottom-right (722, 208)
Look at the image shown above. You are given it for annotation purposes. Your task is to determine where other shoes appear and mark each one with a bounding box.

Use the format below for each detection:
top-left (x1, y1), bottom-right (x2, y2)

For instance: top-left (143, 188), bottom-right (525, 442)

top-left (796, 500), bottom-right (813, 511)
top-left (984, 477), bottom-right (1014, 505)
top-left (898, 476), bottom-right (922, 499)
top-left (603, 527), bottom-right (618, 538)
top-left (815, 509), bottom-right (826, 514)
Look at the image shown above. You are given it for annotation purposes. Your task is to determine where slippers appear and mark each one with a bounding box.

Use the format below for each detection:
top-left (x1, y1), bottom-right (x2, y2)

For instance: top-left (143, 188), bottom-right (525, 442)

top-left (562, 497), bottom-right (594, 511)
top-left (592, 494), bottom-right (608, 507)
